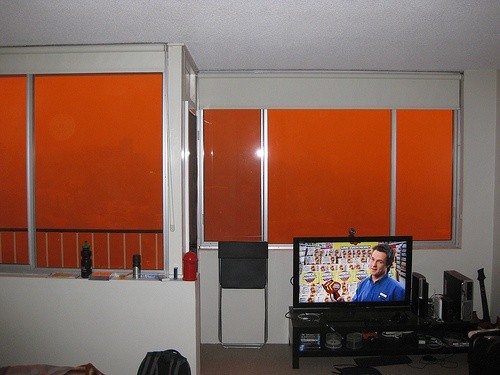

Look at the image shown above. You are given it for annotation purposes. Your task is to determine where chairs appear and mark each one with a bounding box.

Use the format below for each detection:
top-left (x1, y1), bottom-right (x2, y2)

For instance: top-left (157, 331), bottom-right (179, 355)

top-left (216, 240), bottom-right (269, 349)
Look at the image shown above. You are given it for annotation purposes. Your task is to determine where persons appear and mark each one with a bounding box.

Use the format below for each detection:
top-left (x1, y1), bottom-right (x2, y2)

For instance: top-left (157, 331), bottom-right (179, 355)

top-left (331, 244), bottom-right (405, 301)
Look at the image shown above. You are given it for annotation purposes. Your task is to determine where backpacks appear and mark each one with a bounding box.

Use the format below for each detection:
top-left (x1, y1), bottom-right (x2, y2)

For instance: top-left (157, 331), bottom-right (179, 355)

top-left (137, 349), bottom-right (191, 375)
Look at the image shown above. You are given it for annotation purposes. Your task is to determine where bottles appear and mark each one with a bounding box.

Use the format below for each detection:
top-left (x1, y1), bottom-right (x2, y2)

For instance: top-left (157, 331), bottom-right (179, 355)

top-left (80, 241), bottom-right (93, 278)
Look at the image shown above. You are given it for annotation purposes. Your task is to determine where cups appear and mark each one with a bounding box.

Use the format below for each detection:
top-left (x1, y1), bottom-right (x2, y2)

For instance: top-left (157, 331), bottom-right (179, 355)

top-left (325, 333), bottom-right (342, 349)
top-left (346, 333), bottom-right (363, 350)
top-left (133, 255), bottom-right (141, 279)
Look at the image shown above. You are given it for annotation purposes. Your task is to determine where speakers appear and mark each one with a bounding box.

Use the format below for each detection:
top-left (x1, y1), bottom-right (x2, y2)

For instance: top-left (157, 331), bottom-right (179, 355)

top-left (413, 272), bottom-right (429, 324)
top-left (444, 270), bottom-right (473, 322)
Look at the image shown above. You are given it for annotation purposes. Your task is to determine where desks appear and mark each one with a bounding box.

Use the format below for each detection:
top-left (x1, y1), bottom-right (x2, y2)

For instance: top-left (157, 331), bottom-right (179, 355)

top-left (286, 300), bottom-right (422, 369)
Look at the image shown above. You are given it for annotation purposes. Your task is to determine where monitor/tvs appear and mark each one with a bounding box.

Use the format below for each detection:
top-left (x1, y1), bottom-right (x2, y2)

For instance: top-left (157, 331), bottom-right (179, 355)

top-left (294, 236), bottom-right (413, 308)
